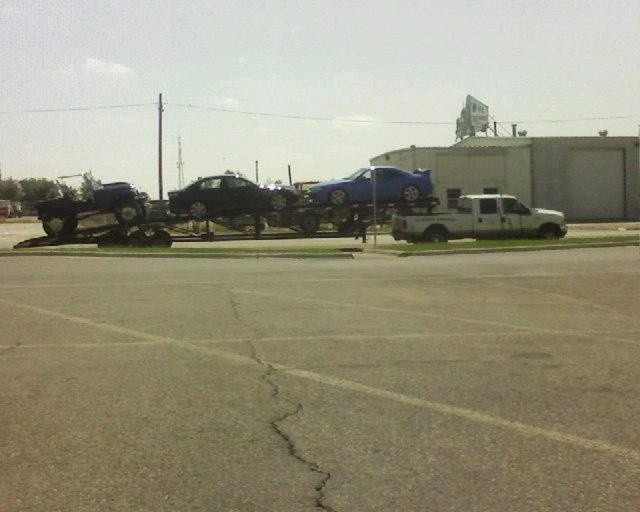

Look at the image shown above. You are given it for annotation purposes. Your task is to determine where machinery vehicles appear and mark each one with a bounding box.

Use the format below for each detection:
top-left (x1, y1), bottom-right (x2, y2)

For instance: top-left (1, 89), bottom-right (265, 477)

top-left (270, 179), bottom-right (346, 234)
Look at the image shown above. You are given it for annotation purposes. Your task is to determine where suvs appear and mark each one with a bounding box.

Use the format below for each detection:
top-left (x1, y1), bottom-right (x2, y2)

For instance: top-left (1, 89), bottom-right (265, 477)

top-left (36, 172), bottom-right (152, 238)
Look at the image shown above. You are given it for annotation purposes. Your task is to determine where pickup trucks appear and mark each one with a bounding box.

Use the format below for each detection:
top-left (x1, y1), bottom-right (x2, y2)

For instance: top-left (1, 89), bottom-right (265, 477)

top-left (390, 191), bottom-right (566, 244)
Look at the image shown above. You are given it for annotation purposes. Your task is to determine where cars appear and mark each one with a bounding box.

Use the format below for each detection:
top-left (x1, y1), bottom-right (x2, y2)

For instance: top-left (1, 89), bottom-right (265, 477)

top-left (170, 173), bottom-right (299, 219)
top-left (309, 165), bottom-right (436, 206)
